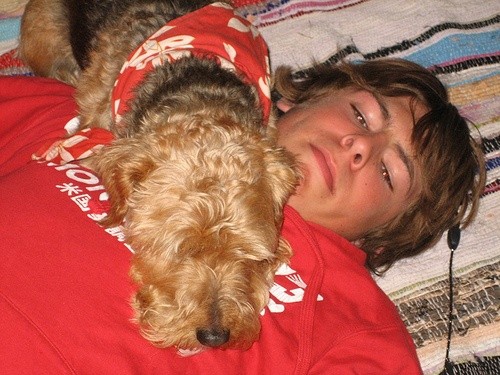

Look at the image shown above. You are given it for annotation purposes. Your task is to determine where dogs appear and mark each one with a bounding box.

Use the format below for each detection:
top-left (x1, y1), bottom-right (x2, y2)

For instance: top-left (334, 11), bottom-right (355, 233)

top-left (15, 0), bottom-right (303, 352)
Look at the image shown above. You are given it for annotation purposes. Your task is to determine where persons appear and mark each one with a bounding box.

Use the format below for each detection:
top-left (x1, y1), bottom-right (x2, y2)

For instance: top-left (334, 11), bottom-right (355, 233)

top-left (1, 60), bottom-right (486, 375)
